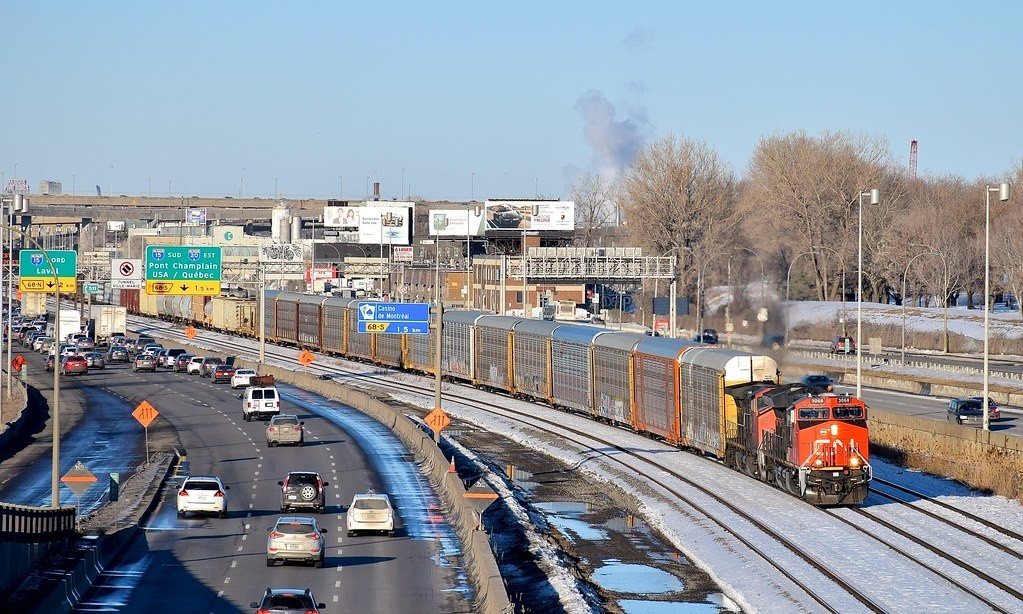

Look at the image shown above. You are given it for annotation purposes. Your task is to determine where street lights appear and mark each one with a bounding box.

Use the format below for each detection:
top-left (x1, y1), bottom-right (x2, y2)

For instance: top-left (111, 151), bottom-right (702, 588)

top-left (467, 206), bottom-right (481, 311)
top-left (813, 246), bottom-right (846, 336)
top-left (901, 253), bottom-right (940, 366)
top-left (523, 205), bottom-right (538, 318)
top-left (380, 213), bottom-right (399, 302)
top-left (348, 245), bottom-right (367, 300)
top-left (297, 243), bottom-right (341, 293)
top-left (784, 252), bottom-right (823, 346)
top-left (857, 189), bottom-right (880, 398)
top-left (700, 252), bottom-right (733, 346)
top-left (733, 247), bottom-right (764, 337)
top-left (982, 182), bottom-right (1011, 432)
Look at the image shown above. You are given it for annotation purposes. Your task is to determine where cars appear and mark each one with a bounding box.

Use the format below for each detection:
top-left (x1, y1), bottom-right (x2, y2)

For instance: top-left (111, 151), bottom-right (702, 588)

top-left (969, 397), bottom-right (1000, 422)
top-left (4, 309), bottom-right (396, 569)
top-left (492, 207), bottom-right (522, 225)
top-left (947, 398), bottom-right (991, 428)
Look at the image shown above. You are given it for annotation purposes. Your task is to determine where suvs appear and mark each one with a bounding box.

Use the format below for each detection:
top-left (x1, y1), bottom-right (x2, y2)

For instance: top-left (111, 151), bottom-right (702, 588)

top-left (829, 336), bottom-right (856, 355)
top-left (277, 471), bottom-right (330, 514)
top-left (250, 587), bottom-right (326, 614)
top-left (694, 329), bottom-right (719, 344)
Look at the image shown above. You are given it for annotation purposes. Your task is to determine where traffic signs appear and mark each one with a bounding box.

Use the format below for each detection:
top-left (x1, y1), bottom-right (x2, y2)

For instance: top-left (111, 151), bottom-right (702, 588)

top-left (18, 250), bottom-right (77, 293)
top-left (145, 245), bottom-right (222, 296)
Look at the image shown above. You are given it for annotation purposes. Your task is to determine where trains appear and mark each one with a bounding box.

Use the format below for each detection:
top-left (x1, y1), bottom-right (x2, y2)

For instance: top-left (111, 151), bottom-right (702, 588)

top-left (59, 271), bottom-right (873, 507)
